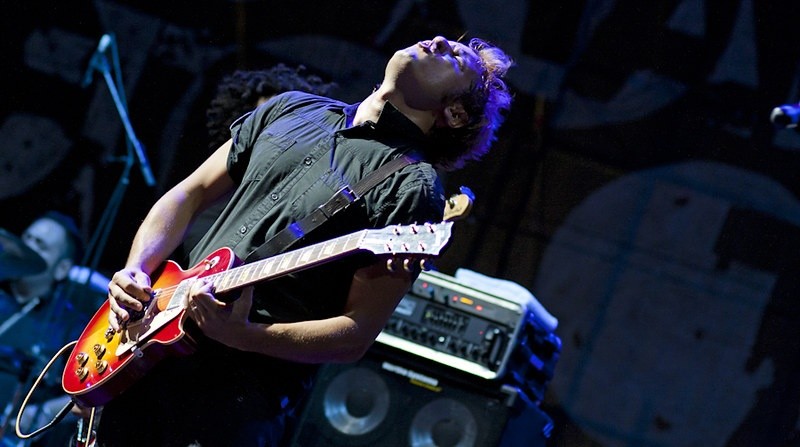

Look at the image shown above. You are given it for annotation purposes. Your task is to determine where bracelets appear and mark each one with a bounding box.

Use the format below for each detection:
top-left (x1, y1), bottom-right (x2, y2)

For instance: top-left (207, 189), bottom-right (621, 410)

top-left (40, 402), bottom-right (53, 421)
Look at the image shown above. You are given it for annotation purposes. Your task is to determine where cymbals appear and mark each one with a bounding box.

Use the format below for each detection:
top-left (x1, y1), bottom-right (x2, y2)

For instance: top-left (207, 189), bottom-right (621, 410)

top-left (0, 229), bottom-right (48, 279)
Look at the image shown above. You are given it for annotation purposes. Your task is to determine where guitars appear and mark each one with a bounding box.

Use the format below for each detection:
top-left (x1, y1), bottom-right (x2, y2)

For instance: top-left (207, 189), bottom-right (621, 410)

top-left (444, 186), bottom-right (476, 221)
top-left (61, 217), bottom-right (456, 408)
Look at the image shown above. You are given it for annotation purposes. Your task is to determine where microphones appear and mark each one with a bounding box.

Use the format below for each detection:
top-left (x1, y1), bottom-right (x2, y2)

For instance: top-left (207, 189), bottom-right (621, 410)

top-left (81, 33), bottom-right (112, 88)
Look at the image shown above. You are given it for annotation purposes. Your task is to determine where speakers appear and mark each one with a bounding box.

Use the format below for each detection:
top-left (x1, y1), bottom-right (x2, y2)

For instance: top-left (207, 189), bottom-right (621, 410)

top-left (290, 345), bottom-right (553, 447)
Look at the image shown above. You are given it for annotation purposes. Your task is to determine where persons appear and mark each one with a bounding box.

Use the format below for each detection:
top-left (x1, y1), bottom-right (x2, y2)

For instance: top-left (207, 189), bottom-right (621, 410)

top-left (95, 35), bottom-right (512, 447)
top-left (177, 63), bottom-right (340, 260)
top-left (0, 212), bottom-right (105, 447)
